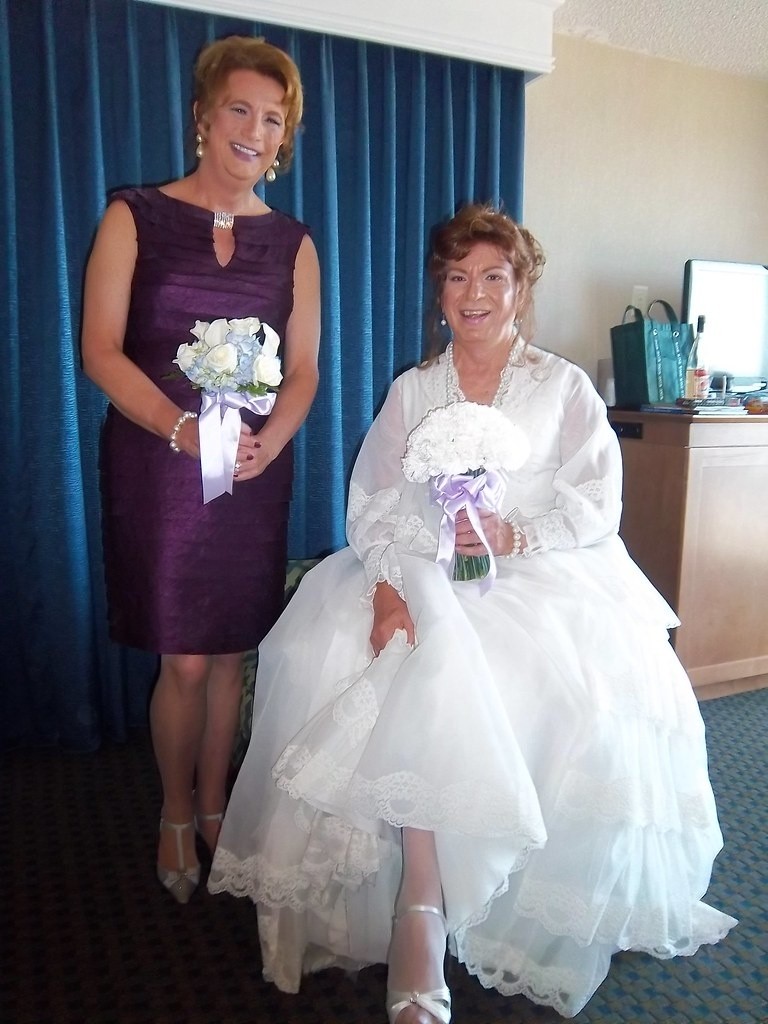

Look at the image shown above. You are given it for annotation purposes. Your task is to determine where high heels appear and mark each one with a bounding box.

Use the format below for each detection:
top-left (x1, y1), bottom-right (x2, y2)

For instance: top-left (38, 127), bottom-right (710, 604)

top-left (156, 807), bottom-right (200, 903)
top-left (195, 812), bottom-right (224, 853)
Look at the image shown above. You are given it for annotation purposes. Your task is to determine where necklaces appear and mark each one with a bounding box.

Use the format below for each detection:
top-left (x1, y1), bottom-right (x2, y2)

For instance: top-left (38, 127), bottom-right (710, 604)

top-left (447, 326), bottom-right (519, 407)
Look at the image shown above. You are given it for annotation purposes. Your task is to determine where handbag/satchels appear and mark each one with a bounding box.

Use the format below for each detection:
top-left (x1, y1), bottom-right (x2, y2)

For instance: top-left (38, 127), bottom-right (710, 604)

top-left (611, 300), bottom-right (696, 409)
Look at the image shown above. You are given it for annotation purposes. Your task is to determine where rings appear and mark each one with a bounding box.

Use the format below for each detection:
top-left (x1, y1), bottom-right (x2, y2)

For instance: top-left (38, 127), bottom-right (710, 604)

top-left (236, 461), bottom-right (241, 468)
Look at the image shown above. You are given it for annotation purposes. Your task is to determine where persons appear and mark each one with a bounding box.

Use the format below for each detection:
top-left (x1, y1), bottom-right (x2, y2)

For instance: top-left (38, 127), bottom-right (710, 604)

top-left (208, 197), bottom-right (740, 1024)
top-left (83, 33), bottom-right (323, 900)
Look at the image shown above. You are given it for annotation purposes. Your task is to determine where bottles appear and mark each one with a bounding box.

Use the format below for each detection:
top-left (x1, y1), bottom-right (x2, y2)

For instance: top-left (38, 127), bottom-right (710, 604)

top-left (684, 315), bottom-right (710, 398)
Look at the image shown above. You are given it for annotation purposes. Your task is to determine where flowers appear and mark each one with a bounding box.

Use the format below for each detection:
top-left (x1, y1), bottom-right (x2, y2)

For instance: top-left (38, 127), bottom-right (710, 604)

top-left (172, 317), bottom-right (284, 397)
top-left (401, 402), bottom-right (531, 582)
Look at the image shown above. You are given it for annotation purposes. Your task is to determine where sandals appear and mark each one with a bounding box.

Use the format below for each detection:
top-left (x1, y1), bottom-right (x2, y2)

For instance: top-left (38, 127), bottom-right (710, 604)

top-left (385, 903), bottom-right (450, 1023)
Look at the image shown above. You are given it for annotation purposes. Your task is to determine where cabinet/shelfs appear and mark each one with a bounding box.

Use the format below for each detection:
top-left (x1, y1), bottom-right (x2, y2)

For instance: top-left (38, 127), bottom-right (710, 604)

top-left (608, 409), bottom-right (768, 702)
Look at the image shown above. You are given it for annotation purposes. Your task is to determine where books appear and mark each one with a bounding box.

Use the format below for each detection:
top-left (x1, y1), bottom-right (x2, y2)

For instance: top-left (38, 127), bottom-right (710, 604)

top-left (639, 397), bottom-right (748, 415)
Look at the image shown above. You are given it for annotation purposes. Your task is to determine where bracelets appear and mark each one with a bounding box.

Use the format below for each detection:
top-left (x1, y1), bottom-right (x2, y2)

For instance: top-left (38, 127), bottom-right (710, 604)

top-left (502, 521), bottom-right (523, 561)
top-left (170, 410), bottom-right (198, 453)
